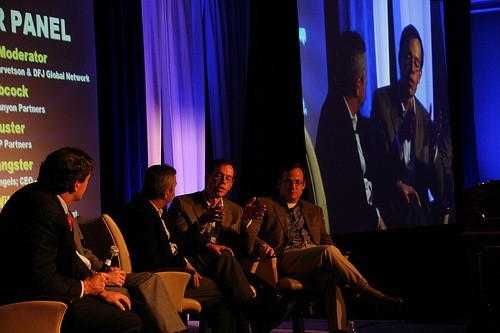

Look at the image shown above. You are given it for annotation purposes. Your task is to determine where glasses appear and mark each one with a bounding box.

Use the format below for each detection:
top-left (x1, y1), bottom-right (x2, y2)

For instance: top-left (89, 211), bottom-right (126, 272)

top-left (213, 172), bottom-right (234, 183)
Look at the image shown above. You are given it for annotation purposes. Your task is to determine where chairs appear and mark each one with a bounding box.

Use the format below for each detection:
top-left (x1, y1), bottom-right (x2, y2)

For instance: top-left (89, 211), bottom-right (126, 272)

top-left (0, 213), bottom-right (350, 333)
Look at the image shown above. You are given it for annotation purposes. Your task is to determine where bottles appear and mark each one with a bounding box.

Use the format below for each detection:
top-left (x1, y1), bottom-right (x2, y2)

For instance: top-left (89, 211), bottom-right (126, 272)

top-left (346, 321), bottom-right (357, 333)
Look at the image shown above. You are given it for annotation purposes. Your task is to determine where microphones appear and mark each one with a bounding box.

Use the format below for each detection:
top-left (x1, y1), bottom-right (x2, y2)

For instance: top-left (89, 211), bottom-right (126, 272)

top-left (99, 245), bottom-right (119, 273)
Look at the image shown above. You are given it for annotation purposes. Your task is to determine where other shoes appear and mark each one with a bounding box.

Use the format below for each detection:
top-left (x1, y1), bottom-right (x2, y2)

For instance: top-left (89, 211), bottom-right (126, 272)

top-left (250, 297), bottom-right (295, 333)
top-left (346, 292), bottom-right (404, 317)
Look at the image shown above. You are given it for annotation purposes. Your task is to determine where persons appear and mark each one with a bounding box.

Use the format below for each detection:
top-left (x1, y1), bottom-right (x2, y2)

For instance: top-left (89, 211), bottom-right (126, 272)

top-left (0, 147), bottom-right (146, 333)
top-left (67, 206), bottom-right (187, 333)
top-left (314, 25), bottom-right (452, 232)
top-left (124, 165), bottom-right (255, 333)
top-left (240, 160), bottom-right (403, 333)
top-left (165, 158), bottom-right (306, 333)
